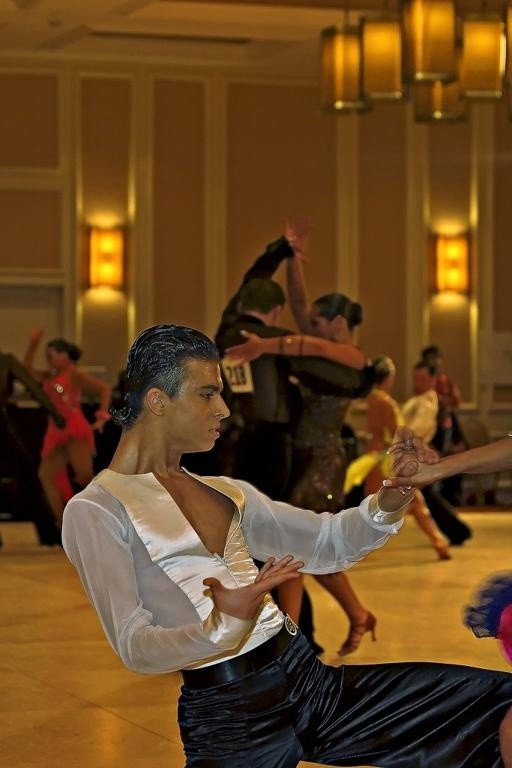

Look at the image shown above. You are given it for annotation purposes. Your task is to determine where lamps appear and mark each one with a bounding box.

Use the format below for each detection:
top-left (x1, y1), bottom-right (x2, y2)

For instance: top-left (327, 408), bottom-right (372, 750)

top-left (320, 0), bottom-right (512, 124)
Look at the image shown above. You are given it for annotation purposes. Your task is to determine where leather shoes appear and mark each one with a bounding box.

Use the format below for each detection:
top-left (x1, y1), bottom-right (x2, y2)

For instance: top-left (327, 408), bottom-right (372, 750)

top-left (40, 525), bottom-right (62, 546)
top-left (433, 530), bottom-right (471, 559)
top-left (303, 632), bottom-right (323, 657)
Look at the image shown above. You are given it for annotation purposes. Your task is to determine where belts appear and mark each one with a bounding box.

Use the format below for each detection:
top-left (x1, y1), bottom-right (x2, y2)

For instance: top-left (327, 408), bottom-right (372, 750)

top-left (180, 612), bottom-right (299, 690)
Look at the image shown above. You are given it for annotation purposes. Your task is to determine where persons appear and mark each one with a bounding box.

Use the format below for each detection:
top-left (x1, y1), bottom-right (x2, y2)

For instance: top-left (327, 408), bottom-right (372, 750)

top-left (381, 436), bottom-right (512, 490)
top-left (61, 323), bottom-right (511, 768)
top-left (1, 221), bottom-right (472, 657)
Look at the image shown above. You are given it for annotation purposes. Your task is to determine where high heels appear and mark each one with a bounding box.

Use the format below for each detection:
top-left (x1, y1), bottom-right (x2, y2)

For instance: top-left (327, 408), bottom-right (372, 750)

top-left (338, 611), bottom-right (376, 655)
